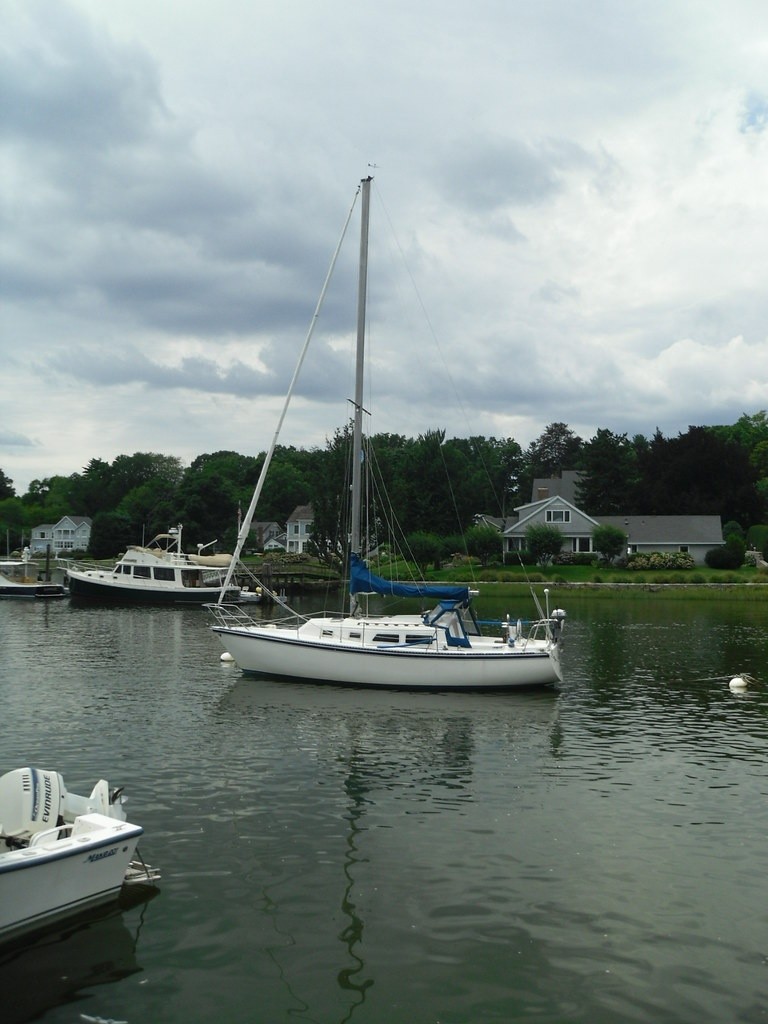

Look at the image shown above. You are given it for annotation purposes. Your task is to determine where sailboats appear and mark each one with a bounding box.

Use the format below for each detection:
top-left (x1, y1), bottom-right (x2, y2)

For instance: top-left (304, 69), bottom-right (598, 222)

top-left (199, 159), bottom-right (572, 688)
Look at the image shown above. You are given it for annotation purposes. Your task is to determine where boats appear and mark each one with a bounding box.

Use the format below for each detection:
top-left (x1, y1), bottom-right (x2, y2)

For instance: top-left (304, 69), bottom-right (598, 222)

top-left (240, 584), bottom-right (288, 604)
top-left (0, 764), bottom-right (166, 935)
top-left (62, 523), bottom-right (244, 606)
